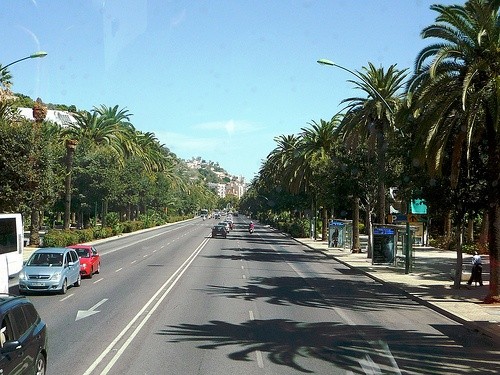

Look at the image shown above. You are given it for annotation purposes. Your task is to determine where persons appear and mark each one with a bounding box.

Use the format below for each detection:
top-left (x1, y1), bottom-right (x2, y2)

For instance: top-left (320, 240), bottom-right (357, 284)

top-left (466, 251), bottom-right (483, 287)
top-left (249, 221), bottom-right (254, 229)
top-left (332, 228), bottom-right (339, 247)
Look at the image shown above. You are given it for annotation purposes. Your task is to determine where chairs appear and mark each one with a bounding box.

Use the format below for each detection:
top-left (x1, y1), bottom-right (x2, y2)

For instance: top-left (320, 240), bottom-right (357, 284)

top-left (39, 254), bottom-right (48, 261)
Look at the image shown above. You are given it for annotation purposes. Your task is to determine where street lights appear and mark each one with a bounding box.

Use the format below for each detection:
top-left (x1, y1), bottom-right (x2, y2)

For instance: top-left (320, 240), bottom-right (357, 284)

top-left (317, 58), bottom-right (413, 274)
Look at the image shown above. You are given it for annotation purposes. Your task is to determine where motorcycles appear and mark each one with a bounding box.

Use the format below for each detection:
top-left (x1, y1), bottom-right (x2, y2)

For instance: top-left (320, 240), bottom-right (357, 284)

top-left (249, 225), bottom-right (254, 234)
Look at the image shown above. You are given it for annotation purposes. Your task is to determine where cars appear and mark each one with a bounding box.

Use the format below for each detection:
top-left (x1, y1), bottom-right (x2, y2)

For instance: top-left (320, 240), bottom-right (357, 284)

top-left (214, 211), bottom-right (239, 233)
top-left (211, 226), bottom-right (227, 238)
top-left (68, 245), bottom-right (101, 279)
top-left (18, 247), bottom-right (81, 295)
top-left (0, 294), bottom-right (47, 375)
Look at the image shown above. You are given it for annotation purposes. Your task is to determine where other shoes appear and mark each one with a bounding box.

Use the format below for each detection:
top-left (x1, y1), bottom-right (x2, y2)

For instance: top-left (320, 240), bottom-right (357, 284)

top-left (480, 284), bottom-right (483, 286)
top-left (467, 283), bottom-right (471, 285)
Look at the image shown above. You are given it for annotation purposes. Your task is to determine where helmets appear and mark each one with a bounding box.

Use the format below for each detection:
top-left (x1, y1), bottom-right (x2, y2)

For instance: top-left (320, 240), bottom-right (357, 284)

top-left (251, 221), bottom-right (252, 223)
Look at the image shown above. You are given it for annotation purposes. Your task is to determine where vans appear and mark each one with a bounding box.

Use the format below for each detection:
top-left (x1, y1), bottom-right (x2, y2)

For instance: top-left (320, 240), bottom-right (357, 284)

top-left (0, 213), bottom-right (23, 279)
top-left (201, 209), bottom-right (208, 217)
